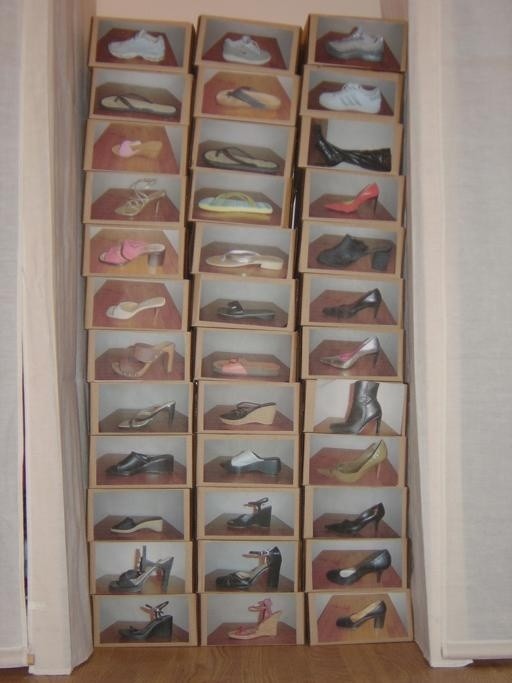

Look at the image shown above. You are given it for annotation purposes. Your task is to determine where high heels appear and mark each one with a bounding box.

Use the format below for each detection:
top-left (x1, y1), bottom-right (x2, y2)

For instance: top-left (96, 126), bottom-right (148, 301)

top-left (106, 297), bottom-right (164, 321)
top-left (99, 240), bottom-right (165, 266)
top-left (325, 503), bottom-right (385, 534)
top-left (328, 549), bottom-right (390, 585)
top-left (112, 141), bottom-right (163, 160)
top-left (118, 401), bottom-right (176, 429)
top-left (227, 498), bottom-right (270, 528)
top-left (318, 235), bottom-right (392, 270)
top-left (228, 599), bottom-right (280, 639)
top-left (114, 179), bottom-right (165, 216)
top-left (321, 336), bottom-right (381, 370)
top-left (325, 183), bottom-right (379, 216)
top-left (109, 545), bottom-right (174, 592)
top-left (337, 600), bottom-right (386, 628)
top-left (112, 341), bottom-right (174, 377)
top-left (217, 546), bottom-right (282, 587)
top-left (323, 288), bottom-right (381, 319)
top-left (332, 439), bottom-right (388, 483)
top-left (119, 601), bottom-right (172, 639)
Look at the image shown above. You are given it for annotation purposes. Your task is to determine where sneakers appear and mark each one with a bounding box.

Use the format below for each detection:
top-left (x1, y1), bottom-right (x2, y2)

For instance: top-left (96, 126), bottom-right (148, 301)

top-left (109, 30), bottom-right (165, 62)
top-left (319, 83), bottom-right (384, 113)
top-left (223, 36), bottom-right (272, 66)
top-left (325, 26), bottom-right (384, 63)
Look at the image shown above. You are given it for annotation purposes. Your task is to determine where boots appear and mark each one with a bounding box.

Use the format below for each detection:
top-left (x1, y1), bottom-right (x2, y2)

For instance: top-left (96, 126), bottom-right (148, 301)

top-left (330, 380), bottom-right (381, 435)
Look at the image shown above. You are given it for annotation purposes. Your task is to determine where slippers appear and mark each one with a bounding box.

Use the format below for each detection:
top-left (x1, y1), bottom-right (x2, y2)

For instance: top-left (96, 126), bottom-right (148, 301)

top-left (212, 357), bottom-right (280, 377)
top-left (204, 146), bottom-right (279, 173)
top-left (198, 191), bottom-right (273, 214)
top-left (206, 250), bottom-right (283, 270)
top-left (216, 86), bottom-right (280, 110)
top-left (218, 302), bottom-right (276, 321)
top-left (102, 92), bottom-right (176, 117)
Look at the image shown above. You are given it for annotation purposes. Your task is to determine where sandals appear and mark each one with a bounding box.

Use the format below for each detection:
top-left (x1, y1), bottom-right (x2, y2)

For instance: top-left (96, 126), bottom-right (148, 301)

top-left (220, 402), bottom-right (277, 425)
top-left (107, 452), bottom-right (173, 475)
top-left (221, 450), bottom-right (281, 474)
top-left (111, 517), bottom-right (162, 533)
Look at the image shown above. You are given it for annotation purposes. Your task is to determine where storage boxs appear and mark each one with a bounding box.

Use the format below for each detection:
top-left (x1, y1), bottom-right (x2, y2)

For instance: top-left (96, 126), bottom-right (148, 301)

top-left (193, 328), bottom-right (296, 381)
top-left (302, 68), bottom-right (400, 112)
top-left (302, 274), bottom-right (402, 327)
top-left (301, 115), bottom-right (400, 174)
top-left (195, 436), bottom-right (300, 485)
top-left (307, 14), bottom-right (406, 67)
top-left (87, 540), bottom-right (190, 594)
top-left (93, 594), bottom-right (194, 649)
top-left (85, 120), bottom-right (191, 174)
top-left (196, 487), bottom-right (299, 540)
top-left (306, 380), bottom-right (406, 434)
top-left (86, 330), bottom-right (190, 382)
top-left (86, 381), bottom-right (193, 434)
top-left (88, 14), bottom-right (192, 69)
top-left (83, 67), bottom-right (192, 120)
top-left (80, 174), bottom-right (186, 224)
top-left (83, 279), bottom-right (187, 329)
top-left (303, 169), bottom-right (402, 221)
top-left (307, 593), bottom-right (413, 645)
top-left (83, 488), bottom-right (192, 542)
top-left (305, 485), bottom-right (405, 539)
top-left (304, 539), bottom-right (406, 592)
top-left (302, 220), bottom-right (401, 274)
top-left (191, 116), bottom-right (296, 179)
top-left (201, 597), bottom-right (301, 646)
top-left (191, 276), bottom-right (295, 331)
top-left (82, 220), bottom-right (186, 279)
top-left (187, 170), bottom-right (292, 227)
top-left (196, 14), bottom-right (303, 72)
top-left (196, 539), bottom-right (297, 596)
top-left (192, 223), bottom-right (295, 281)
top-left (196, 380), bottom-right (298, 433)
top-left (303, 327), bottom-right (402, 382)
top-left (87, 433), bottom-right (193, 489)
top-left (305, 433), bottom-right (405, 484)
top-left (193, 68), bottom-right (298, 126)
